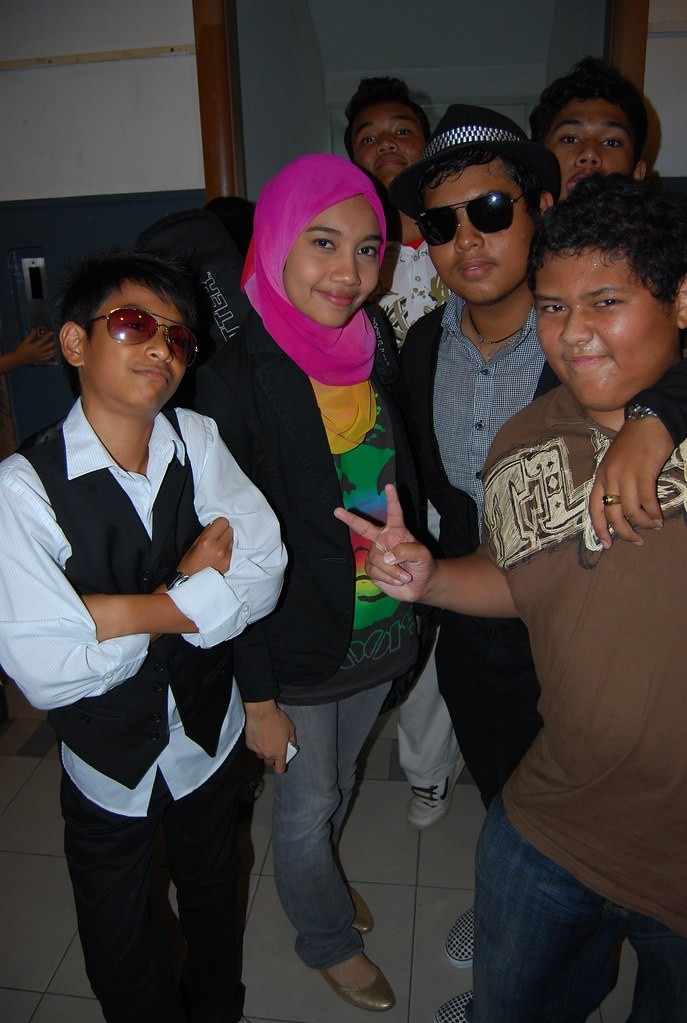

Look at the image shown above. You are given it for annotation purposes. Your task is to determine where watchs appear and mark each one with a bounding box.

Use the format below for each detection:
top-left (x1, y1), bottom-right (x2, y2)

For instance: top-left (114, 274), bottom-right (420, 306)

top-left (624, 404), bottom-right (656, 420)
top-left (164, 570), bottom-right (190, 591)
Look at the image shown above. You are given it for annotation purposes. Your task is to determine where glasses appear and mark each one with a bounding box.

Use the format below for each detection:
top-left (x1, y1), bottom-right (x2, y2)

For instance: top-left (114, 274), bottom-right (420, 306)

top-left (409, 192), bottom-right (525, 247)
top-left (80, 304), bottom-right (198, 366)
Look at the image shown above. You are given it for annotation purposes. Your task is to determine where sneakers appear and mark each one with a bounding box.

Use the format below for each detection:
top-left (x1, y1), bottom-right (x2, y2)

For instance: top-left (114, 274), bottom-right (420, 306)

top-left (444, 904), bottom-right (474, 970)
top-left (406, 752), bottom-right (466, 830)
top-left (435, 988), bottom-right (473, 1023)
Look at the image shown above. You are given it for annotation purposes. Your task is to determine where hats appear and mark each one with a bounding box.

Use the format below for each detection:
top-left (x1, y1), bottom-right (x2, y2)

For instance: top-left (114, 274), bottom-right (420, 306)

top-left (388, 104), bottom-right (562, 223)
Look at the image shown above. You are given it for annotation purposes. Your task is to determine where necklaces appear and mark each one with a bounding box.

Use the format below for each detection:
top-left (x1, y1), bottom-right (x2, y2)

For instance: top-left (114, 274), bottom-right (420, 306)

top-left (467, 303), bottom-right (534, 361)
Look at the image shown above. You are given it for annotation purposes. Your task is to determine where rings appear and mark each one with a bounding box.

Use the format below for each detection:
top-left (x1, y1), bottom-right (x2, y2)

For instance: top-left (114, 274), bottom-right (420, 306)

top-left (601, 494), bottom-right (622, 505)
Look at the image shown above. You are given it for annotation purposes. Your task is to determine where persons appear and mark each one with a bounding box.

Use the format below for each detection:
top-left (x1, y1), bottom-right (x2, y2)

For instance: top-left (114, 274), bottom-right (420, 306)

top-left (333, 102), bottom-right (687, 1022)
top-left (0, 246), bottom-right (289, 1023)
top-left (527, 54), bottom-right (649, 204)
top-left (195, 156), bottom-right (446, 1014)
top-left (332, 169), bottom-right (687, 1023)
top-left (0, 327), bottom-right (59, 462)
top-left (344, 75), bottom-right (466, 827)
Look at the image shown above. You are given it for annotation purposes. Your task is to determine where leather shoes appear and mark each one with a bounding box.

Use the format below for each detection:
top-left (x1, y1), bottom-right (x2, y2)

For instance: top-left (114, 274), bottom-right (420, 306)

top-left (348, 885), bottom-right (375, 933)
top-left (319, 951), bottom-right (397, 1012)
